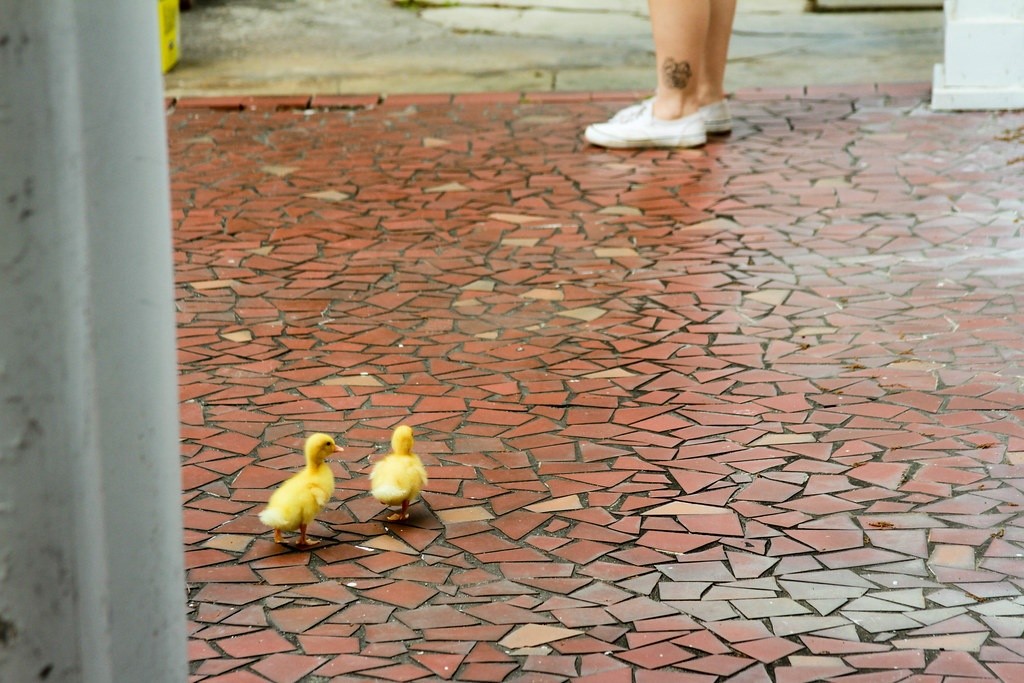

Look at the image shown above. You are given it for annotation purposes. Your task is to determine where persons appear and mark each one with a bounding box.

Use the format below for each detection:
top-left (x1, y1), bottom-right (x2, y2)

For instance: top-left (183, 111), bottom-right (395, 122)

top-left (584, 0), bottom-right (737, 148)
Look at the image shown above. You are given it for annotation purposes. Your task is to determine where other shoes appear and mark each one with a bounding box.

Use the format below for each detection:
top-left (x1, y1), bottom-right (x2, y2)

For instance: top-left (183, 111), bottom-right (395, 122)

top-left (609, 98), bottom-right (731, 132)
top-left (584, 113), bottom-right (707, 148)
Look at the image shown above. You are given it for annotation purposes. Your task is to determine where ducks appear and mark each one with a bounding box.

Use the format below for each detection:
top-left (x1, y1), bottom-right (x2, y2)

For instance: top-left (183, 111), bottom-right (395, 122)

top-left (369, 423), bottom-right (429, 521)
top-left (257, 435), bottom-right (345, 546)
